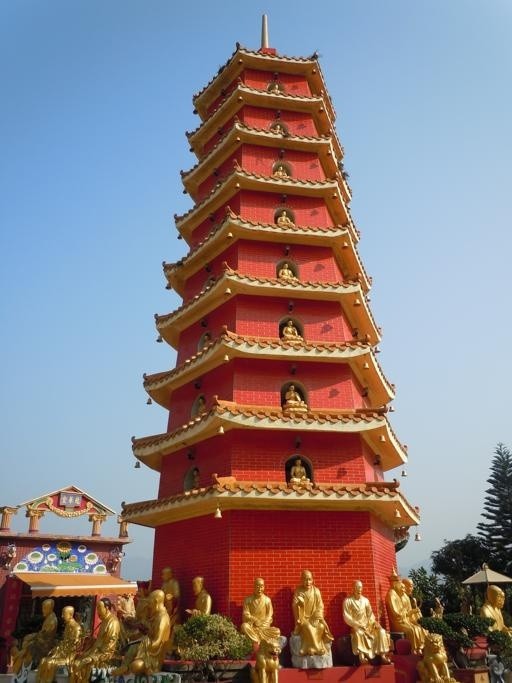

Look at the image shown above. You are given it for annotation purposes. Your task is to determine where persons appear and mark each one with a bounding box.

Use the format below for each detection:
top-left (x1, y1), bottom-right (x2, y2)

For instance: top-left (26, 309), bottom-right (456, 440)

top-left (281, 318), bottom-right (300, 336)
top-left (277, 209), bottom-right (291, 223)
top-left (341, 578), bottom-right (392, 666)
top-left (284, 383), bottom-right (303, 402)
top-left (288, 455), bottom-right (311, 483)
top-left (290, 568), bottom-right (336, 656)
top-left (275, 124), bottom-right (285, 133)
top-left (384, 576), bottom-right (511, 658)
top-left (10, 564), bottom-right (216, 683)
top-left (270, 83), bottom-right (282, 91)
top-left (239, 575), bottom-right (277, 642)
top-left (273, 164), bottom-right (287, 175)
top-left (278, 260), bottom-right (294, 277)
